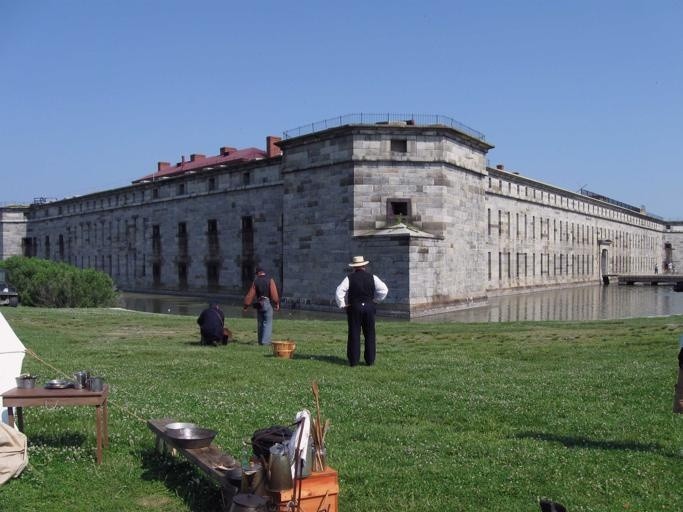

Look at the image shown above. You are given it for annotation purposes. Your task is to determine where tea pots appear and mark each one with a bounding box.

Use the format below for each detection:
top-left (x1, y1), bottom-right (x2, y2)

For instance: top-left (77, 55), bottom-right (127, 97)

top-left (257, 443), bottom-right (300, 494)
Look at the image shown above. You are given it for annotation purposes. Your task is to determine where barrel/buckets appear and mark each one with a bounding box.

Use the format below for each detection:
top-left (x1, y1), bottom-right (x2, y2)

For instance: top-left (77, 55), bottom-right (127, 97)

top-left (270, 341), bottom-right (297, 359)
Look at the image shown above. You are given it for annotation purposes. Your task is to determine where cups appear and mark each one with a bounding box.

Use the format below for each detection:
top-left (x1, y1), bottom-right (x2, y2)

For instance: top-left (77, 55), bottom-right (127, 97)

top-left (311, 445), bottom-right (327, 472)
top-left (72, 369), bottom-right (91, 390)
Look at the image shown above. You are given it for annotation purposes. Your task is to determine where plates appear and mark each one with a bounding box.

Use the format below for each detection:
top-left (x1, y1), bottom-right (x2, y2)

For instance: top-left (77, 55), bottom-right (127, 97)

top-left (44, 379), bottom-right (71, 389)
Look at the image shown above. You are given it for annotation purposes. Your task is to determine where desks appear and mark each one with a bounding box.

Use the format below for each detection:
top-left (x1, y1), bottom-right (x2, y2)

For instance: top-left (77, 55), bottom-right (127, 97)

top-left (0, 384), bottom-right (110, 467)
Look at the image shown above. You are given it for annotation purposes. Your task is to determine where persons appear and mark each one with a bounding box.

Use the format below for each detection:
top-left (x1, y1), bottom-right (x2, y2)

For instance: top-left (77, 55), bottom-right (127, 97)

top-left (240, 265), bottom-right (280, 345)
top-left (196, 299), bottom-right (225, 346)
top-left (334, 254), bottom-right (389, 367)
top-left (655, 264), bottom-right (659, 275)
top-left (670, 345), bottom-right (682, 415)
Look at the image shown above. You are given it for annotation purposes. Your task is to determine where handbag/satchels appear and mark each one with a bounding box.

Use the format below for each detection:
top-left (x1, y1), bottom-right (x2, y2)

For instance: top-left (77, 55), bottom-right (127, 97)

top-left (222, 328), bottom-right (229, 346)
top-left (252, 297), bottom-right (264, 309)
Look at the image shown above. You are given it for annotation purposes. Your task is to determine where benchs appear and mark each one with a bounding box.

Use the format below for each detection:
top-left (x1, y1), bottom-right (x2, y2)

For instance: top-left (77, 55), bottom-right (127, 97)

top-left (147, 418), bottom-right (241, 497)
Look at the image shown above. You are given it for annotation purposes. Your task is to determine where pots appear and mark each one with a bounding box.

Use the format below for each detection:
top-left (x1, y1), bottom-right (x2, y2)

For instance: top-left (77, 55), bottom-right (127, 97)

top-left (15, 375), bottom-right (36, 389)
top-left (229, 489), bottom-right (267, 512)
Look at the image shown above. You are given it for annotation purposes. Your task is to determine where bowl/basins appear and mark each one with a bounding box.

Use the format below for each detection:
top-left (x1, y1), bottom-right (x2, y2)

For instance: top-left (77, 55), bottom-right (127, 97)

top-left (164, 422), bottom-right (194, 431)
top-left (164, 427), bottom-right (217, 447)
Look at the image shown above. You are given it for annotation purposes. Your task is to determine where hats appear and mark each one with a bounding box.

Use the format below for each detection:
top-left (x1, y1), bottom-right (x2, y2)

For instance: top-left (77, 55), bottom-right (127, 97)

top-left (348, 255), bottom-right (370, 267)
